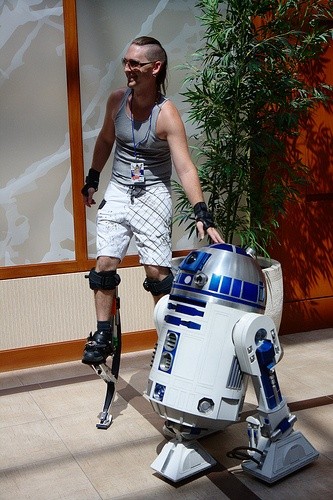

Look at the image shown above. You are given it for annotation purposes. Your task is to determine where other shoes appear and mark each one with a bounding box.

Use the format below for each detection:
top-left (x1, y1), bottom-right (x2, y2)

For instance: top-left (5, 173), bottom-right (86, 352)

top-left (82, 331), bottom-right (114, 365)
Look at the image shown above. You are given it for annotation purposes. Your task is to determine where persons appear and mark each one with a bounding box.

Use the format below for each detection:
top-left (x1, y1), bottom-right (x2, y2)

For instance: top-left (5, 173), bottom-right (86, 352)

top-left (81, 35), bottom-right (225, 365)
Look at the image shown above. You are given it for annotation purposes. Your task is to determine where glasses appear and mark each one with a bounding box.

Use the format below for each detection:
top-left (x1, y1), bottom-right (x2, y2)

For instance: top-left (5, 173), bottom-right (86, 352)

top-left (121, 57), bottom-right (155, 67)
